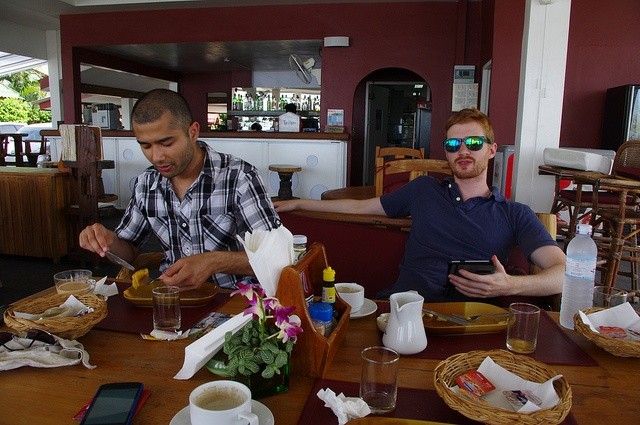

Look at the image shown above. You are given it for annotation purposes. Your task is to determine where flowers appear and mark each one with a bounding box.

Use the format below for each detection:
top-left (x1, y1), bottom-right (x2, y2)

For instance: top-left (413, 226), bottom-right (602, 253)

top-left (213, 282), bottom-right (304, 378)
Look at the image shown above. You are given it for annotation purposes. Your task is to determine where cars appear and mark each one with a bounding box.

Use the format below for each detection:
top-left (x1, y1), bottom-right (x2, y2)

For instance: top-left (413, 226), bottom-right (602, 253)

top-left (2, 123), bottom-right (52, 169)
top-left (0, 122), bottom-right (28, 133)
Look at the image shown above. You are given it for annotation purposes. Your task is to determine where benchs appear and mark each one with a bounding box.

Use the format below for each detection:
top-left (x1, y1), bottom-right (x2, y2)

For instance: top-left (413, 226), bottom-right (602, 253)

top-left (269, 208), bottom-right (557, 314)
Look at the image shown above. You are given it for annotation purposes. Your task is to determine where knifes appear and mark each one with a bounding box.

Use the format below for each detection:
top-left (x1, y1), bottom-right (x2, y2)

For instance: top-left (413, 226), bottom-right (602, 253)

top-left (423, 309), bottom-right (467, 327)
top-left (106, 252), bottom-right (135, 270)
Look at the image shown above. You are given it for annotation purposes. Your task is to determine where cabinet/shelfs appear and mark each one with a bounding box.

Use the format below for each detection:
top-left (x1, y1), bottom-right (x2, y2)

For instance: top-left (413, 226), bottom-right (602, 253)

top-left (227, 81), bottom-right (320, 136)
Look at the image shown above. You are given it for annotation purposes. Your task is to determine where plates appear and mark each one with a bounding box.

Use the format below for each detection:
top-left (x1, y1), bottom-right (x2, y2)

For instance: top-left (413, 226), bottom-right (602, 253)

top-left (169, 399), bottom-right (275, 424)
top-left (420, 302), bottom-right (516, 329)
top-left (350, 297), bottom-right (378, 317)
top-left (124, 280), bottom-right (217, 308)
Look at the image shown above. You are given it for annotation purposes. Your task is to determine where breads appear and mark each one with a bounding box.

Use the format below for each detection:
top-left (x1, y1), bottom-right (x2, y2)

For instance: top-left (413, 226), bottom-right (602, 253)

top-left (130, 269), bottom-right (148, 290)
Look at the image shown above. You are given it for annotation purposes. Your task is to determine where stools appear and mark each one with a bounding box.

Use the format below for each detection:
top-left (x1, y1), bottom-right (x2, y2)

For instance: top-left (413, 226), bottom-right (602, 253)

top-left (267, 164), bottom-right (303, 202)
top-left (62, 161), bottom-right (79, 202)
top-left (95, 160), bottom-right (119, 203)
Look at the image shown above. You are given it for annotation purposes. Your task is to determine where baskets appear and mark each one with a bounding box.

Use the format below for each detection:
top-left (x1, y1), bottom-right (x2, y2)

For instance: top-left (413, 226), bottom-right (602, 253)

top-left (573, 307), bottom-right (640, 358)
top-left (3, 292), bottom-right (108, 341)
top-left (434, 348), bottom-right (573, 425)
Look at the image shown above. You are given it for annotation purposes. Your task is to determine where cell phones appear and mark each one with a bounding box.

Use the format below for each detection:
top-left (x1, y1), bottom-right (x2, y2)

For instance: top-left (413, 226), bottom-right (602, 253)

top-left (448, 260), bottom-right (495, 279)
top-left (80, 382), bottom-right (144, 425)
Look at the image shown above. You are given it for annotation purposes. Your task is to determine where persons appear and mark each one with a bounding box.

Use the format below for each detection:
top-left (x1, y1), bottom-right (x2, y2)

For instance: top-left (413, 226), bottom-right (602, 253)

top-left (270, 107), bottom-right (567, 302)
top-left (79, 88), bottom-right (282, 295)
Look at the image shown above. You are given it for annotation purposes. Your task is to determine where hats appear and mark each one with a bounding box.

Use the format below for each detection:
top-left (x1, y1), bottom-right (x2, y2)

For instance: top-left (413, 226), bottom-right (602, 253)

top-left (283, 104), bottom-right (297, 109)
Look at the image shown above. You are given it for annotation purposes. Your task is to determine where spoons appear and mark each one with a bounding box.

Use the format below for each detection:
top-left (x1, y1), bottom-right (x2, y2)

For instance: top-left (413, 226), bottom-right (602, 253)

top-left (468, 314), bottom-right (509, 321)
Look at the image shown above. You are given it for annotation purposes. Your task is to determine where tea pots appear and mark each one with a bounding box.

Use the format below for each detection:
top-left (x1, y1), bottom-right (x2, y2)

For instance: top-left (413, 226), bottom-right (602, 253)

top-left (382, 290), bottom-right (429, 356)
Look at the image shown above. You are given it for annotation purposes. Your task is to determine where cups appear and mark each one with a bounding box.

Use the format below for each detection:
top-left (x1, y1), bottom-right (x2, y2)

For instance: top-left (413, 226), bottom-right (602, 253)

top-left (593, 286), bottom-right (628, 309)
top-left (334, 282), bottom-right (365, 312)
top-left (359, 346), bottom-right (400, 409)
top-left (188, 380), bottom-right (258, 425)
top-left (505, 302), bottom-right (541, 352)
top-left (152, 285), bottom-right (182, 331)
top-left (53, 270), bottom-right (92, 294)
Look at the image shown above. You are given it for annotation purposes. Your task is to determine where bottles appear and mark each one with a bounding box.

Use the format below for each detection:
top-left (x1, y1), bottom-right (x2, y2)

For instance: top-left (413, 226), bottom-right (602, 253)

top-left (233, 93), bottom-right (242, 110)
top-left (290, 93), bottom-right (300, 110)
top-left (321, 267), bottom-right (335, 304)
top-left (302, 95), bottom-right (320, 111)
top-left (309, 304), bottom-right (333, 334)
top-left (293, 235), bottom-right (307, 261)
top-left (246, 95), bottom-right (263, 111)
top-left (278, 94), bottom-right (288, 110)
top-left (559, 224), bottom-right (597, 328)
top-left (267, 94), bottom-right (277, 111)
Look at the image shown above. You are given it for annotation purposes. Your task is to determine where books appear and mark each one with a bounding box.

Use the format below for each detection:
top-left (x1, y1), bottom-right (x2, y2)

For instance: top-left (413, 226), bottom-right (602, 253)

top-left (327, 109), bottom-right (344, 127)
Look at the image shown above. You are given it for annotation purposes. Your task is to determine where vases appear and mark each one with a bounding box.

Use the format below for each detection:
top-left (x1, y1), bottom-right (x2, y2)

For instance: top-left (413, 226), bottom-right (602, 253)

top-left (207, 358), bottom-right (289, 398)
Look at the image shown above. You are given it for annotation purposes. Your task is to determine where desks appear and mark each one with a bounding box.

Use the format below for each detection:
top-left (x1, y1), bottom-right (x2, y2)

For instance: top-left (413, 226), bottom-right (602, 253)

top-left (320, 184), bottom-right (387, 201)
top-left (6, 153), bottom-right (42, 167)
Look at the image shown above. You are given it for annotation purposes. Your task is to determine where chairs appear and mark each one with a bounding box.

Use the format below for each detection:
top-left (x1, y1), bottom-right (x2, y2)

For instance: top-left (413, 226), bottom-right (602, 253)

top-left (541, 161), bottom-right (634, 256)
top-left (0, 133), bottom-right (28, 166)
top-left (374, 145), bottom-right (425, 181)
top-left (374, 155), bottom-right (452, 199)
top-left (39, 131), bottom-right (64, 160)
top-left (589, 141), bottom-right (639, 307)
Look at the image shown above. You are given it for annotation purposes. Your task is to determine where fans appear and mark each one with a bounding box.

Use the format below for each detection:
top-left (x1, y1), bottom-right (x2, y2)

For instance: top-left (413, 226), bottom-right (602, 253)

top-left (289, 53), bottom-right (316, 84)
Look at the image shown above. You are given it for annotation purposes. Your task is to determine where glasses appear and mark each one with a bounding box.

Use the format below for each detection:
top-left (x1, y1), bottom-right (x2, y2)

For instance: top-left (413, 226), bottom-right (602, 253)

top-left (442, 136), bottom-right (492, 153)
top-left (0, 329), bottom-right (56, 351)
